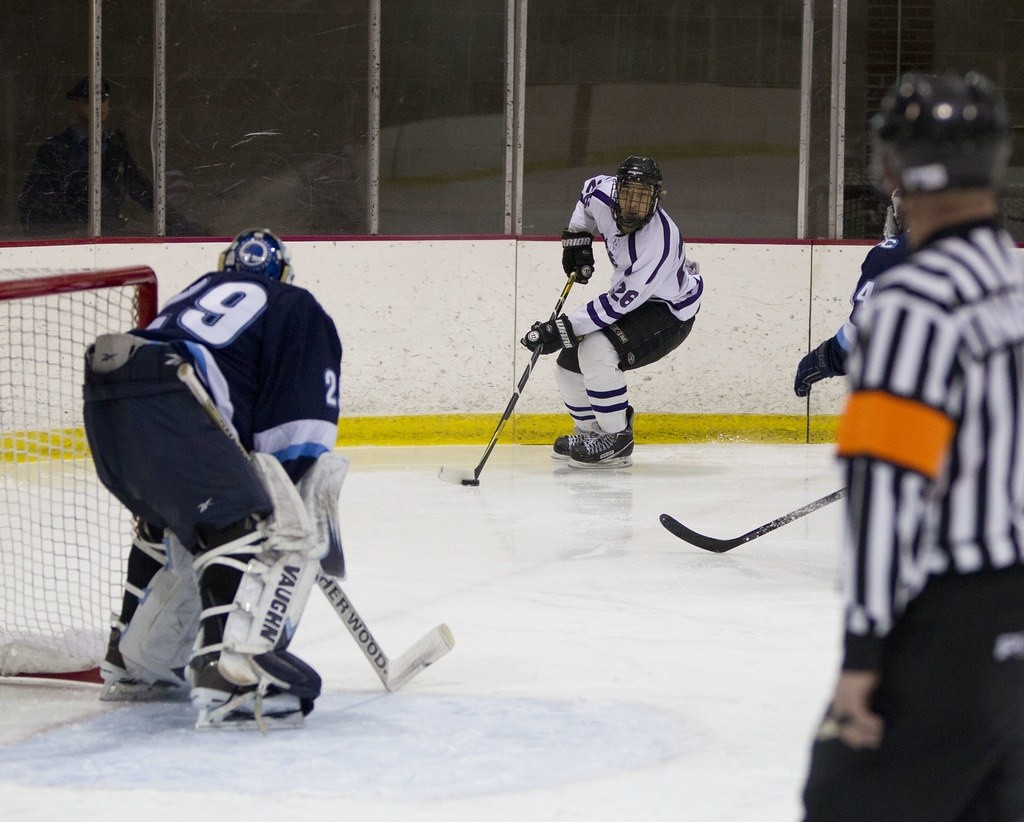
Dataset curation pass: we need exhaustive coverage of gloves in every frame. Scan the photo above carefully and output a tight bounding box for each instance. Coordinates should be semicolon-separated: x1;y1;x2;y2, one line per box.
793;335;846;398
561;227;595;285
520;312;579;355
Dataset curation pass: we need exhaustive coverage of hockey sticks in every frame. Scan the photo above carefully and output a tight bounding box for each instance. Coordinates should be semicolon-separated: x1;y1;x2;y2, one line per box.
438;264;578;484
659;486;848;554
178;361;456;693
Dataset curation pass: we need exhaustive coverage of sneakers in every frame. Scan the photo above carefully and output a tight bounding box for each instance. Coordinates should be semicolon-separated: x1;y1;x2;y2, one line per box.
567;406;634;470
98;638;190;701
551;424;599;460
190;659;301;731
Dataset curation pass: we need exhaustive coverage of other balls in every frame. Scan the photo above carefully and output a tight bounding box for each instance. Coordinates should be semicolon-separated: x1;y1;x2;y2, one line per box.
462;479;480;486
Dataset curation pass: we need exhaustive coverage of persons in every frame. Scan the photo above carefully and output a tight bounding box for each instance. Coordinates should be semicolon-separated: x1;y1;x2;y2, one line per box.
521;155;703;468
794;67;1024;822
20;79;211;235
83;228;341;722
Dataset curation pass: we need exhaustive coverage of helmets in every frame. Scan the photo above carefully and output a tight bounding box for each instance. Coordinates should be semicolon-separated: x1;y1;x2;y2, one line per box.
610;155;663;229
869;66;1010;196
217;228;294;286
